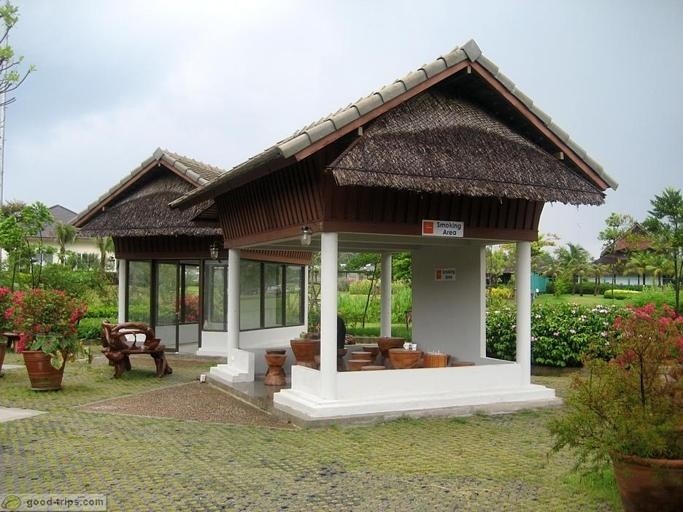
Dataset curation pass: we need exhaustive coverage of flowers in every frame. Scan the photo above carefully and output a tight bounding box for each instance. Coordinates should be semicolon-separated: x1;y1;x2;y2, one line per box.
0;287;15;344
7;286;91;374
548;298;683;482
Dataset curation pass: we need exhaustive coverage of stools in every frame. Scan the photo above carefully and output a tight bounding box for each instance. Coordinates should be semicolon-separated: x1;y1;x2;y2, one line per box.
262;333;475;394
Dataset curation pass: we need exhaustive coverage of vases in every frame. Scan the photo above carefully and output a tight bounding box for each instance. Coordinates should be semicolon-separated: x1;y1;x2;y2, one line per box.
19;348;65;388
608;454;683;511
0;343;8;377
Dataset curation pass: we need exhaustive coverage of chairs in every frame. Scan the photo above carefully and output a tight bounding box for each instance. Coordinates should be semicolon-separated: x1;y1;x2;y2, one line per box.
100;318;173;379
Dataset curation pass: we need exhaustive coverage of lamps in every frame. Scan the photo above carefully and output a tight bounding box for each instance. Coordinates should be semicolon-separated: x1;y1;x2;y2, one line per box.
209;235;222;264
300;216;317;246
